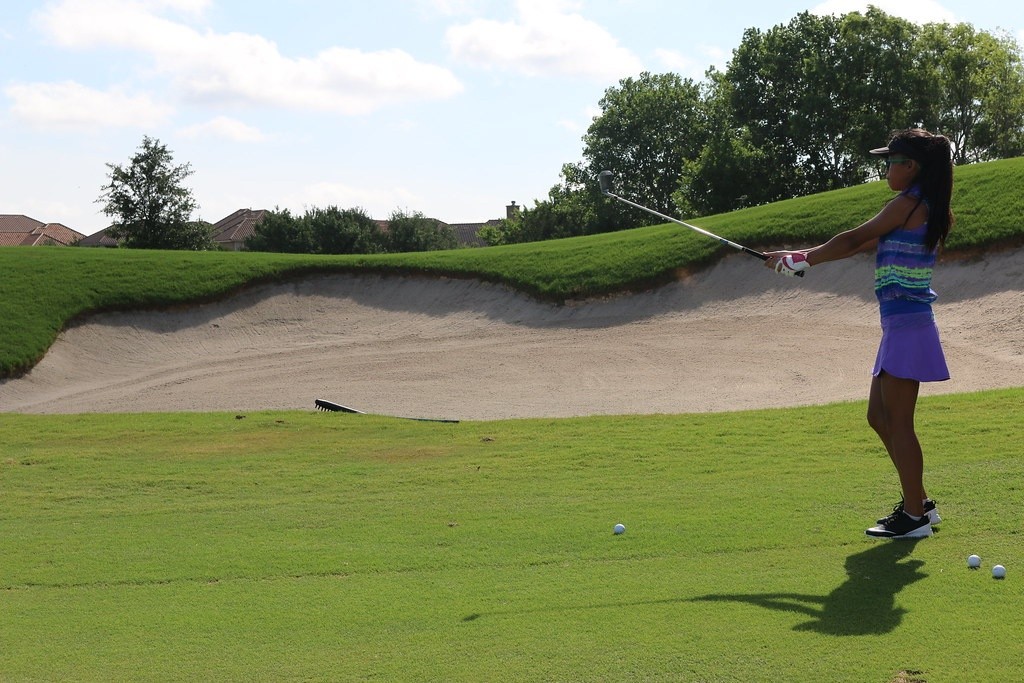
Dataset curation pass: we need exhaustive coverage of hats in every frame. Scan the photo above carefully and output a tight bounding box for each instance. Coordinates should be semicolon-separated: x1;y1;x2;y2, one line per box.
869;136;931;155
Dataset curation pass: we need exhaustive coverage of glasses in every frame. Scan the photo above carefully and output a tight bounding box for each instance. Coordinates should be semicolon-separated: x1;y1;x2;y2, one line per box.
884;158;910;165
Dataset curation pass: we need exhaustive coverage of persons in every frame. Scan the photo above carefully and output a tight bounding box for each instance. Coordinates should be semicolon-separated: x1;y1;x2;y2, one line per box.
763;128;953;538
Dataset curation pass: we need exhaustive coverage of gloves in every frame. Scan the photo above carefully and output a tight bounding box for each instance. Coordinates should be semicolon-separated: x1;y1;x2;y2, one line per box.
775;252;813;277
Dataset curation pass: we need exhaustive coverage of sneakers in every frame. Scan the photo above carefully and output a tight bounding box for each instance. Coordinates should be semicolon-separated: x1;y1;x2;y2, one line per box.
865;510;932;538
876;499;943;526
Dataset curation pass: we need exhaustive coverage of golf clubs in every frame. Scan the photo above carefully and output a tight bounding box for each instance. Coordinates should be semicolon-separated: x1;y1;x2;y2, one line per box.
599;170;805;277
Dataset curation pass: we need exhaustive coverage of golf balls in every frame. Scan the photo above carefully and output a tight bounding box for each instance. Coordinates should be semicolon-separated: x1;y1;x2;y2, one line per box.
992;565;1006;579
613;524;625;534
968;554;981;567
